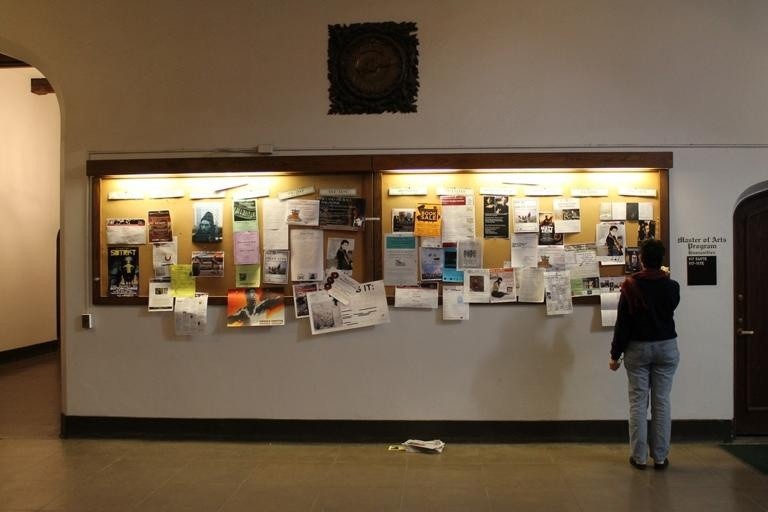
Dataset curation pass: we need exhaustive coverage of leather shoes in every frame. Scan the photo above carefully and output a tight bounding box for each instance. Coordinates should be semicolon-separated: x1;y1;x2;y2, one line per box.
629;457;668;470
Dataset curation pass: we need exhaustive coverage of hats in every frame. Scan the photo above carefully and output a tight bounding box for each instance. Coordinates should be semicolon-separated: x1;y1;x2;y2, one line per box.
201;212;213;225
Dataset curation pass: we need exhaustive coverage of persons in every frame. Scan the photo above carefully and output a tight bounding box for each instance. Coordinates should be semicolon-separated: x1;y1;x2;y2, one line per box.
496;197;508;213
336;240;352;276
192;212;222;241
606;225;623;256
484;197;494;213
491;277;502;296
609;238;681;471
